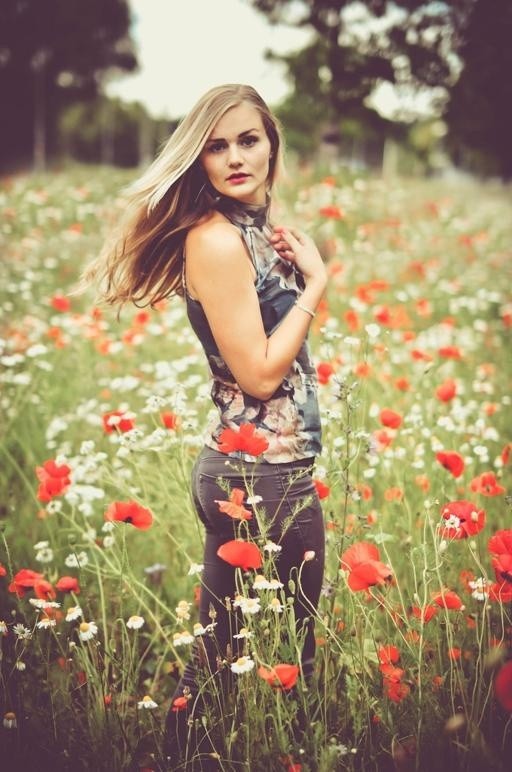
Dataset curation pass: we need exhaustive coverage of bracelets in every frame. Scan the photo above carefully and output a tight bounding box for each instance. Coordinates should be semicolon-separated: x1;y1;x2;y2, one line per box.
292;298;317;319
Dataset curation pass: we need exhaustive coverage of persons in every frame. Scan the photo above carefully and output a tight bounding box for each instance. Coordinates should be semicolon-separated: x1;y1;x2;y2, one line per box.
64;82;330;761
